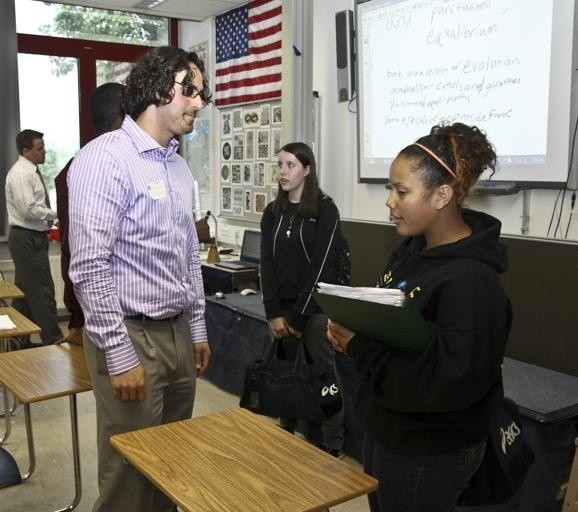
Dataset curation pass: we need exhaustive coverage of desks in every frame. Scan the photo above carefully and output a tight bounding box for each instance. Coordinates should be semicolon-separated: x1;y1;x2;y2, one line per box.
195;249;260;294
111;409;381;510
0;283;25;304
1;342;94;511
1;307;41;416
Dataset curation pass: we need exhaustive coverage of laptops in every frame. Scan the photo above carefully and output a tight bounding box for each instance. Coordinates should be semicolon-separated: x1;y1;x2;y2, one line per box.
215;229;262;271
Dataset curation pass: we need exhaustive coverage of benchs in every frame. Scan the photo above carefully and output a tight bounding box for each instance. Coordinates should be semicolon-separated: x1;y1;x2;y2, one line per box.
195;219;577;511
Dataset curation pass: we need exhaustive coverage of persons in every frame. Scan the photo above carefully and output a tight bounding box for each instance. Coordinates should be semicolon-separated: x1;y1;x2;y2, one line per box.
4;129;64;352
326;122;512;512
259;142;346;459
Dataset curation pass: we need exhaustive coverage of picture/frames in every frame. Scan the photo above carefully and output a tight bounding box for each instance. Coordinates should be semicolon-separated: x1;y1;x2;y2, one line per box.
218;104;282;217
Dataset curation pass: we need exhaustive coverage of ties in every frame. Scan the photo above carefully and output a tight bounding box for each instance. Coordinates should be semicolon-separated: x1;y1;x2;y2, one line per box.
36;165;53;227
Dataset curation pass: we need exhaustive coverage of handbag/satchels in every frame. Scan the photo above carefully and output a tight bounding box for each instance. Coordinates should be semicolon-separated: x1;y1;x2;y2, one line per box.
239;338;343;422
457;396;534;507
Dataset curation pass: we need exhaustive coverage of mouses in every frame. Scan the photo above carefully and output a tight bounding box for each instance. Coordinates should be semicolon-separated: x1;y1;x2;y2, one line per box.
241;289;257;295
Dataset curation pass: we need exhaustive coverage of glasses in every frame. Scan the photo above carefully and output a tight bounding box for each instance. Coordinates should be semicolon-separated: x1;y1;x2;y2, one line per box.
174;81;208;101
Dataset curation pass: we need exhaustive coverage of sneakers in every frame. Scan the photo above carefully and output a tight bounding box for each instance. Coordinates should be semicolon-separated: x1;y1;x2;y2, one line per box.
327;448;346;460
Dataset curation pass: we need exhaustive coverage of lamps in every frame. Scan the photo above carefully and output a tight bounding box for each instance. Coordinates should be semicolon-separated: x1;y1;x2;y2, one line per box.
195;209;224;263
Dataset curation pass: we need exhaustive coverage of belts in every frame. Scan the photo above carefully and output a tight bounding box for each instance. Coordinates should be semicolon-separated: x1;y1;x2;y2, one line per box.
124;309;183;321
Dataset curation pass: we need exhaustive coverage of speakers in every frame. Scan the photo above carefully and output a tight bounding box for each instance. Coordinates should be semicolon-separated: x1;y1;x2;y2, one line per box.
333;8;355;104
